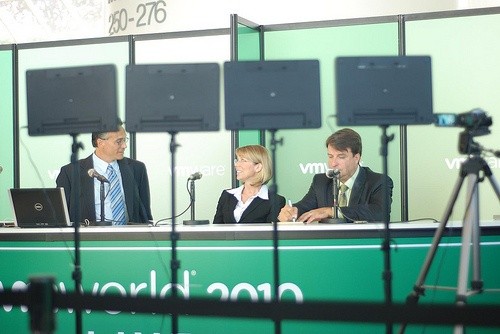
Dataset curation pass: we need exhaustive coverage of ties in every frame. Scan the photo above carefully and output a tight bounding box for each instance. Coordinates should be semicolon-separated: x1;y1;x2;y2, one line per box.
337;184;348;219
107;163;126;225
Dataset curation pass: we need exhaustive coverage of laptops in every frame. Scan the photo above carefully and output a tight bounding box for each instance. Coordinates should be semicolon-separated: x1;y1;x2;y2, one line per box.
9;187;85;228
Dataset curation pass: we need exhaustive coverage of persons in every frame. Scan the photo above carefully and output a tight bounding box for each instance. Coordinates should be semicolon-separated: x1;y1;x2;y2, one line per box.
277;128;394;224
212;145;286;223
56;118;154;228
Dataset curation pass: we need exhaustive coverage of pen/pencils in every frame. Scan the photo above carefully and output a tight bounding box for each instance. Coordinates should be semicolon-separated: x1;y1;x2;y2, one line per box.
288;200;296;222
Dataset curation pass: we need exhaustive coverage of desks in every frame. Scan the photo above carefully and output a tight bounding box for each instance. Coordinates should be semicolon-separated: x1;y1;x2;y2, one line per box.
0;222;500;334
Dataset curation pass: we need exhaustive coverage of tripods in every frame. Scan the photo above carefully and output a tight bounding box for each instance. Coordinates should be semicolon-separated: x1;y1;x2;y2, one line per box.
396;155;500;334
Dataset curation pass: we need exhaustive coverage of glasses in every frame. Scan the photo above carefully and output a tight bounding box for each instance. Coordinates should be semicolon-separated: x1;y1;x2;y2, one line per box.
98;137;128;146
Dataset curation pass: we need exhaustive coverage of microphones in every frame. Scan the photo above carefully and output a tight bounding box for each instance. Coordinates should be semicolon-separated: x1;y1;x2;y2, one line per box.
326;168;340;179
87;169;109;183
189;171;202;180
